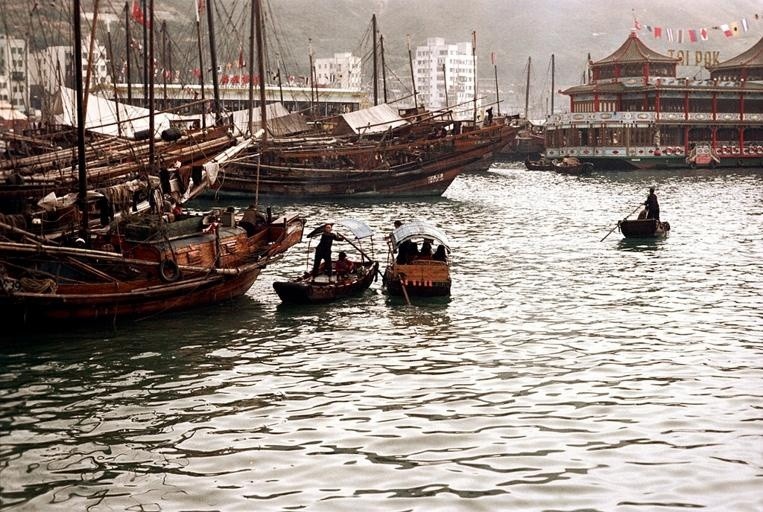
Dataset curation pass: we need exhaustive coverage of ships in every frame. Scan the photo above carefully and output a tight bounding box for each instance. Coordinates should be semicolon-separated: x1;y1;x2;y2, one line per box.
542;31;763;170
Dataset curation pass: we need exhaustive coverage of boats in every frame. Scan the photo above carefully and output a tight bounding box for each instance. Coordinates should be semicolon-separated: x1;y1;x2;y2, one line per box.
525;156;552;171
551;158;594;174
617;219;671;238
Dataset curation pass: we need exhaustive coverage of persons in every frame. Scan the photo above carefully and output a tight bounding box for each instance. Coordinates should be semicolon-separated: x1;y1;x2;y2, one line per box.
395;240;414;264
334;250;352;282
383;219;419;256
225;205;237;228
638;187;659;220
420;242;431;256
310;222;344;284
431;244;447;261
238;204;267;238
201;213;217;238
172;203;191;221
636;205;649;219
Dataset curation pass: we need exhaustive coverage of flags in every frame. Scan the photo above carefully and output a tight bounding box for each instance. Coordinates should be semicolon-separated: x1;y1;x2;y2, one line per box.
128;37;259;89
195;0;206;25
131;1;150;29
633;9;762;46
